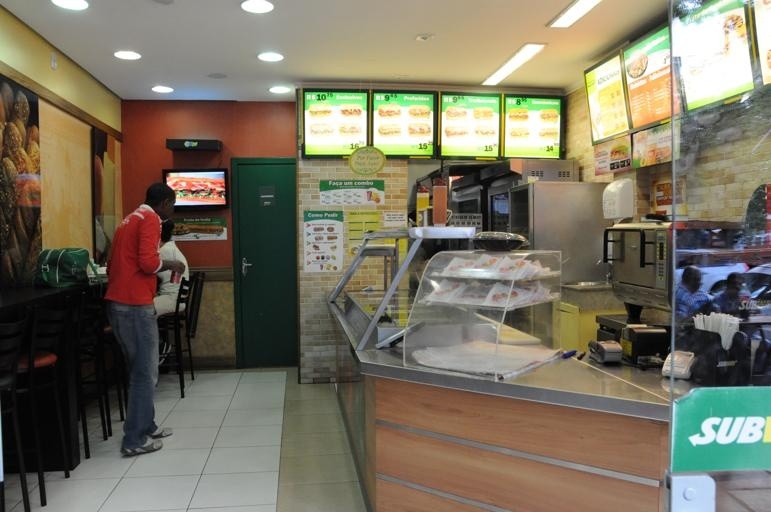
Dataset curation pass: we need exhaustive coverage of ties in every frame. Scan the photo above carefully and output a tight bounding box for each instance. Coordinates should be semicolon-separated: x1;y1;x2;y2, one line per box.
163;168;230;210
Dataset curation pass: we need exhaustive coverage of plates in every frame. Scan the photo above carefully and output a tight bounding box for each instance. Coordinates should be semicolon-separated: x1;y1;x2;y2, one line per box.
328;226;560;381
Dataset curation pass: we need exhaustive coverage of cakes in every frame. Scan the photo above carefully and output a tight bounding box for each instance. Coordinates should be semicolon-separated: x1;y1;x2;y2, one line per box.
169;270;182;284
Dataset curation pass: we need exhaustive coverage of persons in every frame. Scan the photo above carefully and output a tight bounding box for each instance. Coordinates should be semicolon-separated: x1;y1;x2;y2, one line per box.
714;270;770;386
151;219;190;388
674;265;721;386
101;183;185;457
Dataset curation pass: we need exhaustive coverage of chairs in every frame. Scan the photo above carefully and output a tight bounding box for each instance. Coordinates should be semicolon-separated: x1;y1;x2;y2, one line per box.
120;436;162;457
144;425;175;440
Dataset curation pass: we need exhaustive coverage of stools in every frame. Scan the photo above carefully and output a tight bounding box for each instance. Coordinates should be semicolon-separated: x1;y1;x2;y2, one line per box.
0;263;166;459
158;270;205;398
9;303;70;506
2;314;31;512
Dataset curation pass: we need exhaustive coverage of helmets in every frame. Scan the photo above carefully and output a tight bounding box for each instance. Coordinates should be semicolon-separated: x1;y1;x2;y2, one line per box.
627;55;648;79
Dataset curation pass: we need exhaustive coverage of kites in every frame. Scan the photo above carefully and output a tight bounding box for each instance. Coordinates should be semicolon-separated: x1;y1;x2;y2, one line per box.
563;350;577;358
577;351;586;359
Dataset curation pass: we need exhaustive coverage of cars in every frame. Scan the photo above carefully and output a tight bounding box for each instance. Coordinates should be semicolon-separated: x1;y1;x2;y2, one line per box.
646;144;657;166
415;184;448;227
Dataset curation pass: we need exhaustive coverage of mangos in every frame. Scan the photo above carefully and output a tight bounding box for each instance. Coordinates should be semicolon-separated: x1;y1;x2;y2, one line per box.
166;177;226;204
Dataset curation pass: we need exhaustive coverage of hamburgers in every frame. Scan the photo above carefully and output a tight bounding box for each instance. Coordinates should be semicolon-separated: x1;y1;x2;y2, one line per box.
308;102;559;138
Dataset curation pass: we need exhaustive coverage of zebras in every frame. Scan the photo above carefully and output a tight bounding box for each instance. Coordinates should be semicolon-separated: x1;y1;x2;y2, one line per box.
602;220;743;312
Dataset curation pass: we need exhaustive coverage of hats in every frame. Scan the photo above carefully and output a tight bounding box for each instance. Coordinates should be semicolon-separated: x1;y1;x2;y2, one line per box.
674;248;770;353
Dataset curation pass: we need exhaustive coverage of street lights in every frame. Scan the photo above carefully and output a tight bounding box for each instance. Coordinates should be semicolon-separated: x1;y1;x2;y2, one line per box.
35;245;102;291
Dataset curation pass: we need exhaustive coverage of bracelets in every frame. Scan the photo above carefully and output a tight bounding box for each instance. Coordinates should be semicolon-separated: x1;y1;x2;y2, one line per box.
472;238;524;252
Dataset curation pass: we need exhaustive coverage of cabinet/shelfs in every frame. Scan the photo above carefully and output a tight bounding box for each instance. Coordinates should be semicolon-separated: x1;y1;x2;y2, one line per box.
328;226;560;381
